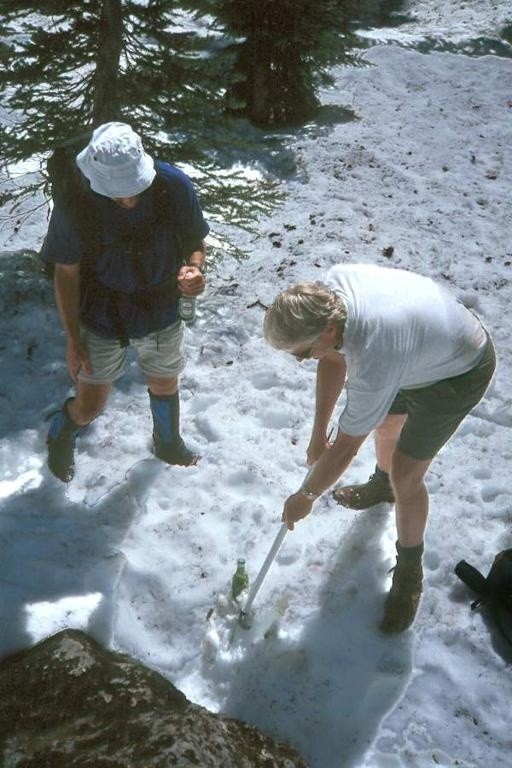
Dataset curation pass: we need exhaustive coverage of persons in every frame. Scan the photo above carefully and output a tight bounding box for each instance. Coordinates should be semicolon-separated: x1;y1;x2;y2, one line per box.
36;118;211;482
266;264;495;635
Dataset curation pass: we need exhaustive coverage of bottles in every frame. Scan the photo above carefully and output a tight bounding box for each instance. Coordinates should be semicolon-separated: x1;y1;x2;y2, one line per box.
230;559;251;602
176;273;197;325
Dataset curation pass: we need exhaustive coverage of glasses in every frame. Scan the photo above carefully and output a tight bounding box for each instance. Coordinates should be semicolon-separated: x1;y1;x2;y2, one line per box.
292;342;315;360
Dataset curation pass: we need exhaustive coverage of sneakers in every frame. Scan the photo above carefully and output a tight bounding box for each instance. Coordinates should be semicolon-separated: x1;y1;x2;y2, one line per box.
334;482;397;510
46;433;76;483
380;568;425;637
152;438;201;466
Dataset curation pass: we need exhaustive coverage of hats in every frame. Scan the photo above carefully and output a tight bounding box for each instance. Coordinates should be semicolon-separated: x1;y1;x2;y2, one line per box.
75;118;157;197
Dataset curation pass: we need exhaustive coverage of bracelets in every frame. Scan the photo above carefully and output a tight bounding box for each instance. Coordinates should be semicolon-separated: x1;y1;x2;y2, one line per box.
300;483;318;501
189;261;206;274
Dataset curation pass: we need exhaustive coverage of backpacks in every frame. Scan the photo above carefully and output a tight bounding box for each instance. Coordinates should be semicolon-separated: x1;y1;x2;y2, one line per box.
455;546;511;637
